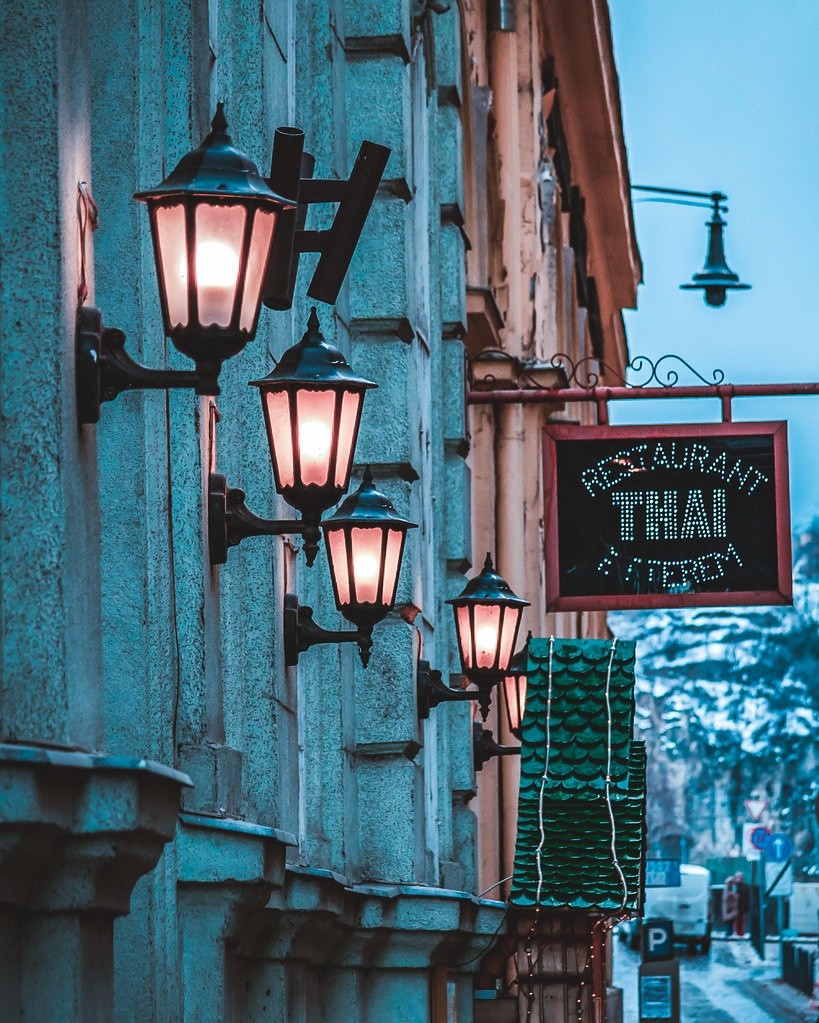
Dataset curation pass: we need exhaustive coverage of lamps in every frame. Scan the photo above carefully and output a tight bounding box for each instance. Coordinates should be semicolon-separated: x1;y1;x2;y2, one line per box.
417;551;529;720
72;99;305;427
629;183;751;308
472;630;529;772
285;462;420;673
206;305;375;573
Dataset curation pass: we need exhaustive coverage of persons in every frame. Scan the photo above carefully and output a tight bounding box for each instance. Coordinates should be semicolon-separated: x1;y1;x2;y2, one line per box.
722;871;750;936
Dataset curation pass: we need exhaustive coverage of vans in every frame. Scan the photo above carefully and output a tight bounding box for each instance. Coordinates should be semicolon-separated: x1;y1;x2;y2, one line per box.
616;863;713;957
